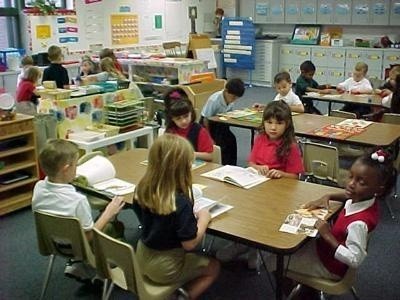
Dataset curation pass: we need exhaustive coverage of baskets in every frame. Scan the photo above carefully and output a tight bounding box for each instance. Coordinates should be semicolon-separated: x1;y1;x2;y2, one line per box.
89;80;130;92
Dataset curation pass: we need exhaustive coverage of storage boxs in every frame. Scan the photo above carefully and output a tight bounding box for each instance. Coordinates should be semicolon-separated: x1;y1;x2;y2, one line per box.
0;47;27;70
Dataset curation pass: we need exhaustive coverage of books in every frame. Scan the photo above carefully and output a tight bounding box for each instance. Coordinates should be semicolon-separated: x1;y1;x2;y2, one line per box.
72;155;137;196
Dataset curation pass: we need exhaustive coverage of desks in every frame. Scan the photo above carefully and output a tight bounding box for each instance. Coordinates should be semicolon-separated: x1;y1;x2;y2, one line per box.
71;148;344;299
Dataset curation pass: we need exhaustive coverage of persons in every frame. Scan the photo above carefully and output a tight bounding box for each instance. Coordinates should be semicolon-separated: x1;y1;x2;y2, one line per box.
133;133;221;300
31;139;126;285
15;45;144;103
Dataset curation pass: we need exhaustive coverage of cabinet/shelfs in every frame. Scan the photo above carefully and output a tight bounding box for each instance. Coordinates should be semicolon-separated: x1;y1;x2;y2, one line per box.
0;72;18;94
0;111;41;215
66;66;80;82
251;1;400;28
278;43;400;89
177;76;228;124
78;57;210;96
19;7;80;52
209;39;224;77
226;37;288;87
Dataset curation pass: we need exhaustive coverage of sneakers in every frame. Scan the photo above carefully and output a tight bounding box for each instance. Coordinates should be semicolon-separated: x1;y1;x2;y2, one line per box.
248;246;261;269
64;257;91;283
215;241;249;262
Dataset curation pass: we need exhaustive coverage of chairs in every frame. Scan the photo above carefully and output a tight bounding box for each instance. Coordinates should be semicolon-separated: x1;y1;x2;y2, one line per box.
92;227;184;300
207;87;399;220
76;151;109;210
33;210;108;299
283;232;371;299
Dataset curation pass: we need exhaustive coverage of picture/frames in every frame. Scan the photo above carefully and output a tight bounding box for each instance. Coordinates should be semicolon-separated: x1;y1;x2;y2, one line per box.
291;21;321;45
